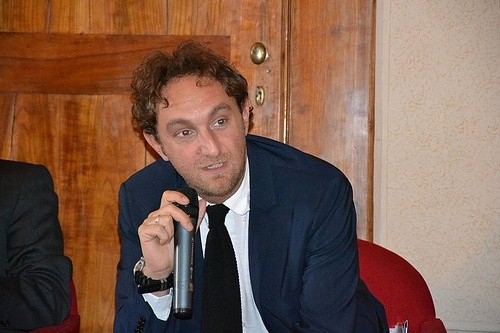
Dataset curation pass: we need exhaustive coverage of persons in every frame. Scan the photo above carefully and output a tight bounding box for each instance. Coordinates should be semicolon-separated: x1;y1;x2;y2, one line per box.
113;42;389;333
0;158;73;333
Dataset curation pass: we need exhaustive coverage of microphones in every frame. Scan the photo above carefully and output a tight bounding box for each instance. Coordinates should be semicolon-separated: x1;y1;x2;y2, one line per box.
172;188;200;321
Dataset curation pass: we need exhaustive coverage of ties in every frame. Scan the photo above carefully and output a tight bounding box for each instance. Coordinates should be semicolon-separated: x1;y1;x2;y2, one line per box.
201;204;243;333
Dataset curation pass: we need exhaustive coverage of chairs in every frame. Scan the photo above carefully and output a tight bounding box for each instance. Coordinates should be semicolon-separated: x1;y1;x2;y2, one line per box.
356;236;447;333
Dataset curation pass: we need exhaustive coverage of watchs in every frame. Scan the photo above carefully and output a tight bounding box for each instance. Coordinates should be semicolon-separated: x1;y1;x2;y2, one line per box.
133;256;175;294
